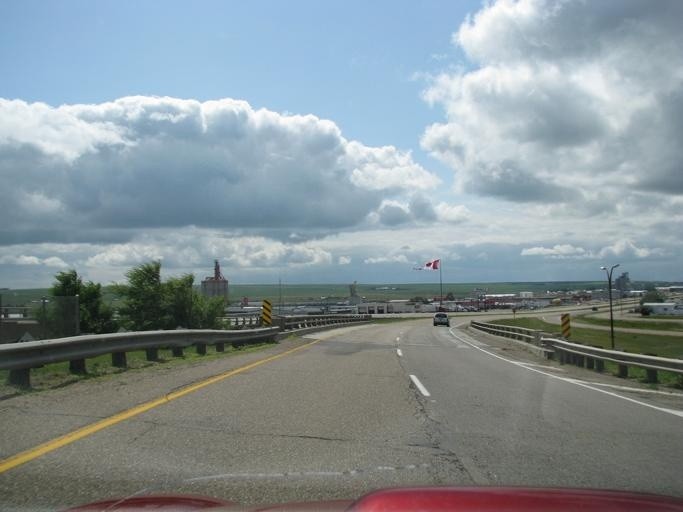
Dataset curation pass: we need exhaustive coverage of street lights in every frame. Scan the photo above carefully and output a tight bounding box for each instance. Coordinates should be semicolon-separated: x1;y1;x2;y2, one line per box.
599;264;619;348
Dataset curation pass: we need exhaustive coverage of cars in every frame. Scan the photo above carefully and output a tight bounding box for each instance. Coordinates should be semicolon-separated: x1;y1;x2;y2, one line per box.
433;312;449;326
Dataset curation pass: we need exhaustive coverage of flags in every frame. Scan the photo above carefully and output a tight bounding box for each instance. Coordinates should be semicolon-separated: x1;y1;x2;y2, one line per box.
412;258;440;271
